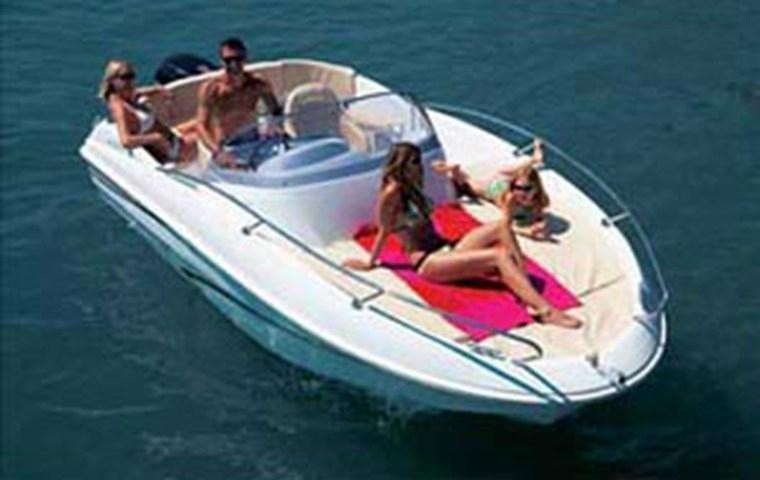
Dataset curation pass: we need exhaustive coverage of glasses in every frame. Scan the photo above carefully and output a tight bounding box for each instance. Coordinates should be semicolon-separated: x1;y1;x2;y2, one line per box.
120;74;134;80
514;185;532;191
224;56;243;63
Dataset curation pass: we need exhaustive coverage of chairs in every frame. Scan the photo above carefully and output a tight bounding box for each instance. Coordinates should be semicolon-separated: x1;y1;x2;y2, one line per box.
282;81;372;158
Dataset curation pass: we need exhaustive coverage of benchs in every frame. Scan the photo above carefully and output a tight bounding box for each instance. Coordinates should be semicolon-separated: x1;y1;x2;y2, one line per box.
136;57;357;167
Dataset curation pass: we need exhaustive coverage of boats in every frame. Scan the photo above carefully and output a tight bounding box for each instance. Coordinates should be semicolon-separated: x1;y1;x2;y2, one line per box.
78;48;669;428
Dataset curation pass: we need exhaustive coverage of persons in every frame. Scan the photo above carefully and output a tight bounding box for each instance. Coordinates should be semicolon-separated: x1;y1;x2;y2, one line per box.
98;59;201;169
196;36;290;174
431;138;552;239
344;141;584;327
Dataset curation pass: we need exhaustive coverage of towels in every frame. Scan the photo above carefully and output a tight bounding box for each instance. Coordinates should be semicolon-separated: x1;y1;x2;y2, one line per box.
350;199;583;342
